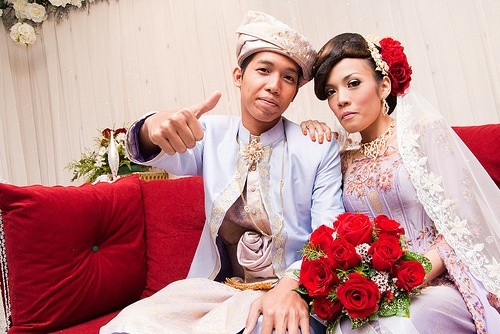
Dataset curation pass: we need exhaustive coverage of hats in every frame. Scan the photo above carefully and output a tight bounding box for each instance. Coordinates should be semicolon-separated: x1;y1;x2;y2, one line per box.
236;9;318;87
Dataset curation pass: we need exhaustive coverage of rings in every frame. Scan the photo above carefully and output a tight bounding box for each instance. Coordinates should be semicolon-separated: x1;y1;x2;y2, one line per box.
307;123;315;128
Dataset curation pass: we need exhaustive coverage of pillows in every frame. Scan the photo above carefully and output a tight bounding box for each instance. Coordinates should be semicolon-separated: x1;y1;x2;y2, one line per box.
0;174;147;334
139;176;207;300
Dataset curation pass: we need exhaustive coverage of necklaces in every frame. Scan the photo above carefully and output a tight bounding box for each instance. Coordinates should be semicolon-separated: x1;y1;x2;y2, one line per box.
237;139;288;238
359;120;395;159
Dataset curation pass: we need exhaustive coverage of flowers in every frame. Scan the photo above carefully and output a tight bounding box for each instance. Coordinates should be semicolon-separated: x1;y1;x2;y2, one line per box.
0;0;96;48
67;121;136;184
367;37;412;97
292;212;433;329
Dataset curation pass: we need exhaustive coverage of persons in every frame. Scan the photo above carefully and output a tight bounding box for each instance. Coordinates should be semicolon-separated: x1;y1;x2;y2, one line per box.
99;11;345;334
299;33;500;334
